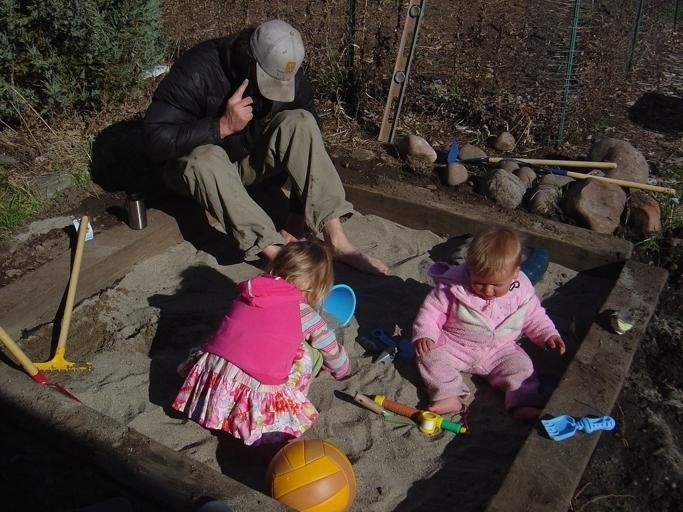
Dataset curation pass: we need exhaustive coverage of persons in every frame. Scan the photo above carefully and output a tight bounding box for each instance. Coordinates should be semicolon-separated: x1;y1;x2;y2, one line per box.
170;234;351;447
143;17;390;277
411;223;567;418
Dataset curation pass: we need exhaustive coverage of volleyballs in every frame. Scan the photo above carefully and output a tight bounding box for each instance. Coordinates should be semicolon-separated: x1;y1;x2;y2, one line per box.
263;439;356;512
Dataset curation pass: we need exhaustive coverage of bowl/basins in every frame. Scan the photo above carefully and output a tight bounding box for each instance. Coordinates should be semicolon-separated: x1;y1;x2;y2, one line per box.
322;284;355;329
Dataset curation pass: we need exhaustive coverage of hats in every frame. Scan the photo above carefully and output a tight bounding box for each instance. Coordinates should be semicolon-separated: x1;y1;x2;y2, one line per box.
247;19;307;105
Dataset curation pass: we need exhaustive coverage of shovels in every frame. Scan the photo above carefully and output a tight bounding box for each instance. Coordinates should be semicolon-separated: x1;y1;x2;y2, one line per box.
353;391;418;426
0;326;83;404
540;413;617;440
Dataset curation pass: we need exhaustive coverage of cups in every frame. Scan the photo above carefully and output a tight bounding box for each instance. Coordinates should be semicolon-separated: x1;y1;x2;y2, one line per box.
126;194;148;231
